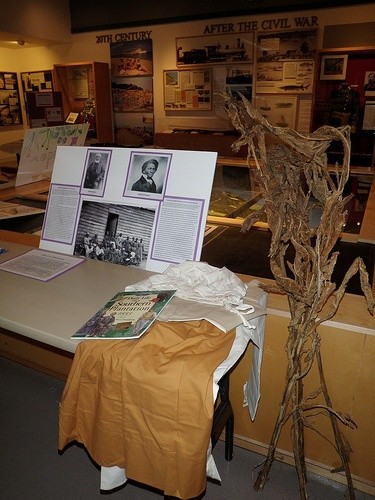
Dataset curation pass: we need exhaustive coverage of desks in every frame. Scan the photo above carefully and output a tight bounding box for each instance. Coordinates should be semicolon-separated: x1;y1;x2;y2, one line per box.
0;239;234;462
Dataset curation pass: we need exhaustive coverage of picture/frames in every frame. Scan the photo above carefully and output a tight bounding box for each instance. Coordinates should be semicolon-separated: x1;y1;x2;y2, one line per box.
254;26;319;96
320;55;347;80
175;31;253;68
162;68;212;112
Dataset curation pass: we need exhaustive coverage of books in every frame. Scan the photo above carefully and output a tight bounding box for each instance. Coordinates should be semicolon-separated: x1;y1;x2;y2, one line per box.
71;290;178;339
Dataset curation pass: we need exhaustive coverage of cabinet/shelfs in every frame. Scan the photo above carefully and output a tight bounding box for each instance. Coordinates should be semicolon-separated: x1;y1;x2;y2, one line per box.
52;61;113;144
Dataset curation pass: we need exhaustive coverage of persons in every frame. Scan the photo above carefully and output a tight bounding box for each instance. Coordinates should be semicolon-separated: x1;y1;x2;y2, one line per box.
78;153;159;266
365;73;375;91
